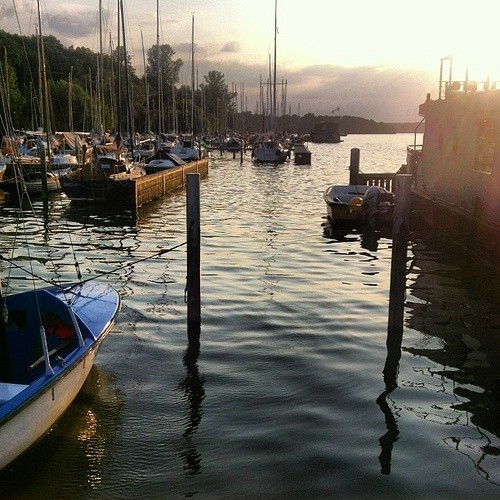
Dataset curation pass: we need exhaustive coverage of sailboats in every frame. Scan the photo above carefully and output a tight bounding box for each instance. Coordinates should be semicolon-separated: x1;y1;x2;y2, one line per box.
1;0;342;464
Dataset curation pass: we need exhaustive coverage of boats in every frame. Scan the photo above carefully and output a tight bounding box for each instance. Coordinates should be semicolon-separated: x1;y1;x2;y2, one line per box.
395;48;500;288
322;189;396;234
